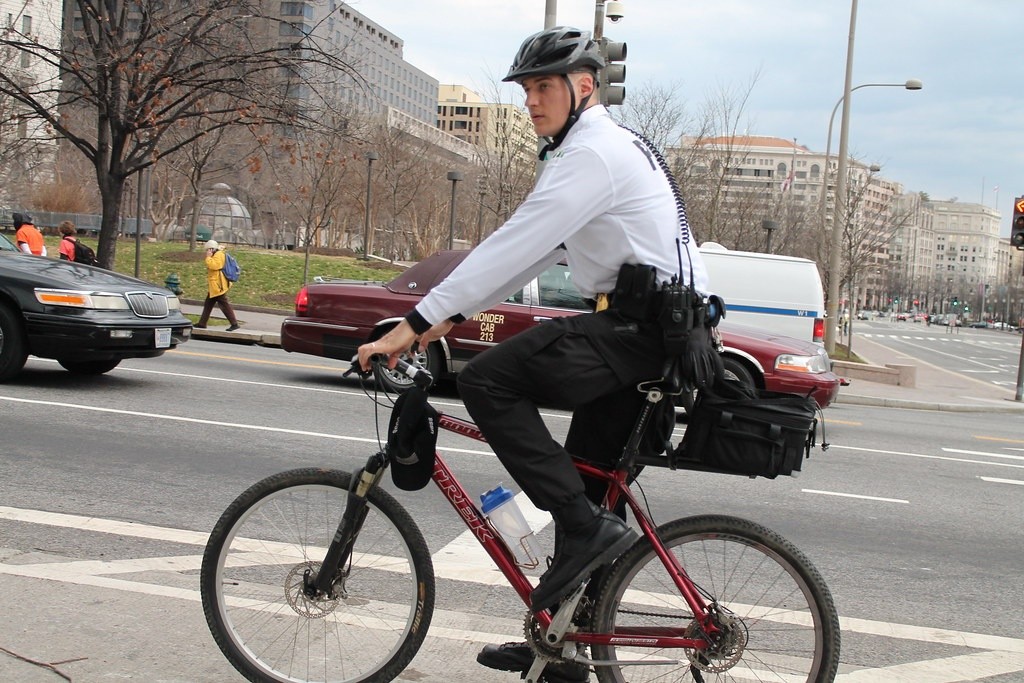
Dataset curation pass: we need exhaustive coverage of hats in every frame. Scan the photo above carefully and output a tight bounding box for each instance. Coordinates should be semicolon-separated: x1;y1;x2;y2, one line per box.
388;386;440;492
205;240;219;249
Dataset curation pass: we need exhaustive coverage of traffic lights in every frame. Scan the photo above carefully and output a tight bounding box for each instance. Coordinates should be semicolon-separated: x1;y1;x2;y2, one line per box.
1010;198;1024;247
914;299;918;305
954;297;957;305
895;297;898;304
888;296;892;302
600;37;628;107
964;305;968;312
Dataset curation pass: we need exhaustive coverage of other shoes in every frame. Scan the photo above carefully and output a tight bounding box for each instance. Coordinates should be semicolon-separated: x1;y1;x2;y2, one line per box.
226;324;242;331
193;323;207;329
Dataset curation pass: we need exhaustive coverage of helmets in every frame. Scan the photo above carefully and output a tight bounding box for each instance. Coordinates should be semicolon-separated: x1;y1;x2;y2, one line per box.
502;25;606;82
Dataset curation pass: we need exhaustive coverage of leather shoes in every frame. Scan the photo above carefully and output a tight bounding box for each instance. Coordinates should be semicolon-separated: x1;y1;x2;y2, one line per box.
529;493;640;612
477;641;590;683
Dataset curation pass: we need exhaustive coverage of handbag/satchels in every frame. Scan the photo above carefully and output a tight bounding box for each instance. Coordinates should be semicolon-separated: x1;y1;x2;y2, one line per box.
675;376;830;480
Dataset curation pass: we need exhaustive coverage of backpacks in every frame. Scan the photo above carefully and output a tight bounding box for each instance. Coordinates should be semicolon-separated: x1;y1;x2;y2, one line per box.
64;238;102;265
221;251;241;282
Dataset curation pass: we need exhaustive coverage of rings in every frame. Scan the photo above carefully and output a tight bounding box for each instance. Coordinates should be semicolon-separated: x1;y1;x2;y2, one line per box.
370;343;375;348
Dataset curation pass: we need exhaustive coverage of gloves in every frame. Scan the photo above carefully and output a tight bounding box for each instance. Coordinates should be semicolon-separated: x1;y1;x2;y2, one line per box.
662;305;725;392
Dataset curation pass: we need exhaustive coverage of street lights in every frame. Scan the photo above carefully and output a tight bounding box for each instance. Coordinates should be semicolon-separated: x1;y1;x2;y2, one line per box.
820;80;923;244
762;218;778;254
128;155;147;277
447;171;465;250
363;152;378;262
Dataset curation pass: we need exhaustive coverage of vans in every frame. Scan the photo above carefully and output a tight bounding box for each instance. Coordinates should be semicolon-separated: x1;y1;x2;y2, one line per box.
692;249;827;345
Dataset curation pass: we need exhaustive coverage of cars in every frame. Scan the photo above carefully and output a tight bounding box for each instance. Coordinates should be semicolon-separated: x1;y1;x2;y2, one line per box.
899;315;906;321
969;321;990;328
858;312;868;320
991;322;1014;329
914;317;921;322
281;251;836;412
930;315;961;326
0;233;193;382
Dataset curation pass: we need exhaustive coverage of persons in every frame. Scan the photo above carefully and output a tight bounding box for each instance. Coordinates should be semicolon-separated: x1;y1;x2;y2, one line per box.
838;317;844;336
57;220;78;262
192;239;241;332
926;314;932;327
843;309;849;336
12;212;47;257
355;25;712;683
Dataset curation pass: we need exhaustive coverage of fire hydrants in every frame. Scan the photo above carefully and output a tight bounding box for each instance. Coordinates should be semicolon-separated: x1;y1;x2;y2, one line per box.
163;272;183;298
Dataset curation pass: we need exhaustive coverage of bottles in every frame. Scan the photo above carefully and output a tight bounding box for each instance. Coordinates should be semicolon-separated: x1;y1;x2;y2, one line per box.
478;485;543;563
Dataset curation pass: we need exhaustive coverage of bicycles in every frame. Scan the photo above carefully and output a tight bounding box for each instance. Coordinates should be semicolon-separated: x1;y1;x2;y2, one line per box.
199;344;841;683
